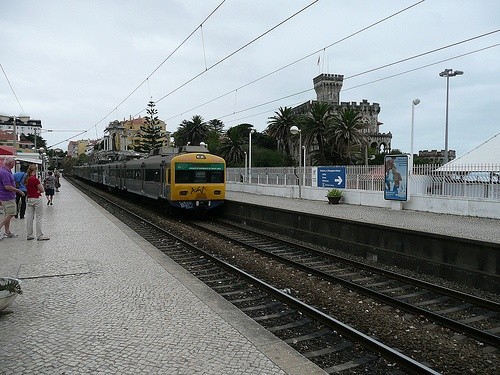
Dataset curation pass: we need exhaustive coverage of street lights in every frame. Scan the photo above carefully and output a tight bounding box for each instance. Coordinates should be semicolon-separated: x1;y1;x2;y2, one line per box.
40;147;49;182
302;146;306;186
439;69;463;163
249;130;254;183
411;99;420;177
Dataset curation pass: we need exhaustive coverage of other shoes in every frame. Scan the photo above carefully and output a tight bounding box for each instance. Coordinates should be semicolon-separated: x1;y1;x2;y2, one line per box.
4;231;18;238
26;236;35;240
0;231;3;240
37;236;50;241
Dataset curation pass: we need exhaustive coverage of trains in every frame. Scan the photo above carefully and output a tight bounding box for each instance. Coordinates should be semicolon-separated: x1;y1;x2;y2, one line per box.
72;146;226;213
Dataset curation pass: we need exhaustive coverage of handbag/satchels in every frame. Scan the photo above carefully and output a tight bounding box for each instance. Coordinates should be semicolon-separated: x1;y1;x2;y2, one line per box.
15;182;20;188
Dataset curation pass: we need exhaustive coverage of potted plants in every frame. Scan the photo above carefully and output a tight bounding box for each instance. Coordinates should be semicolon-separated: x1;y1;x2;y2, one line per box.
0;277;23;311
326;188;343;205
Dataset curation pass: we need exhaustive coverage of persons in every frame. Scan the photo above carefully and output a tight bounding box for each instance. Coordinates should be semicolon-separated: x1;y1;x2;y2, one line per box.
44;171;58;205
13;164;29;220
386;157;402;192
54;170;61;192
23;165;50;240
0;157;25;239
